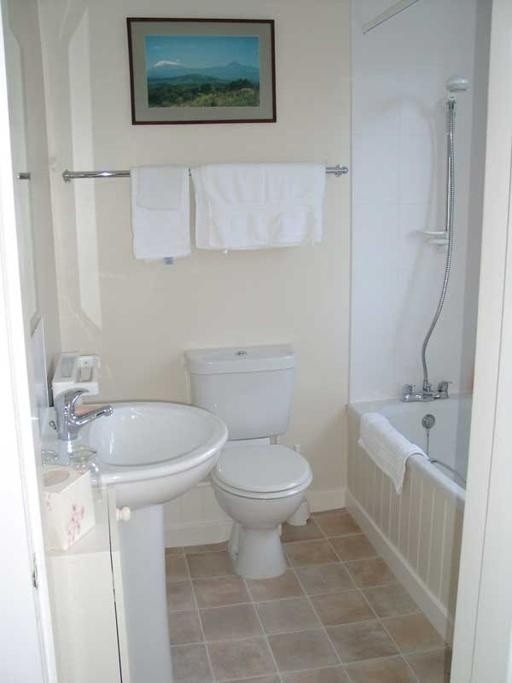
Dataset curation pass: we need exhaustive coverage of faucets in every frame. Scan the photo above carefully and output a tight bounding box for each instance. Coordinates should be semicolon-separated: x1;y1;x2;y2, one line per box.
51;386;113;439
421;381;442;403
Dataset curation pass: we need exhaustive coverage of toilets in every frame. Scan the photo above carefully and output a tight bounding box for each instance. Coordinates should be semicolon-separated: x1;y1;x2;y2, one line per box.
187;347;312;580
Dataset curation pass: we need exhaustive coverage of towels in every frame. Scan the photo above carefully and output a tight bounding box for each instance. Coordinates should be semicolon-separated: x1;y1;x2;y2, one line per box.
192;163;327;251
356;410;429;493
129;164;192;265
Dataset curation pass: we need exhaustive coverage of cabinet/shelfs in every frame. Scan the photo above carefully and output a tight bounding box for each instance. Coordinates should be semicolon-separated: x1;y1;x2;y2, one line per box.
46;486;130;683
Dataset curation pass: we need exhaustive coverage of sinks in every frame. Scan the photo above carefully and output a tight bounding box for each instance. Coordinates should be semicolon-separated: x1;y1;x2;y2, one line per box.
44;401;229;511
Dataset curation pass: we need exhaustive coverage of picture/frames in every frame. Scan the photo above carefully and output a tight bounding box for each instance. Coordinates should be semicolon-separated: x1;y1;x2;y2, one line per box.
127;17;277;124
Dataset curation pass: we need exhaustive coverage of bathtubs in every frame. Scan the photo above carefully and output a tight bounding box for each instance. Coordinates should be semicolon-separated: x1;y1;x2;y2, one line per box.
346;393;469;514
